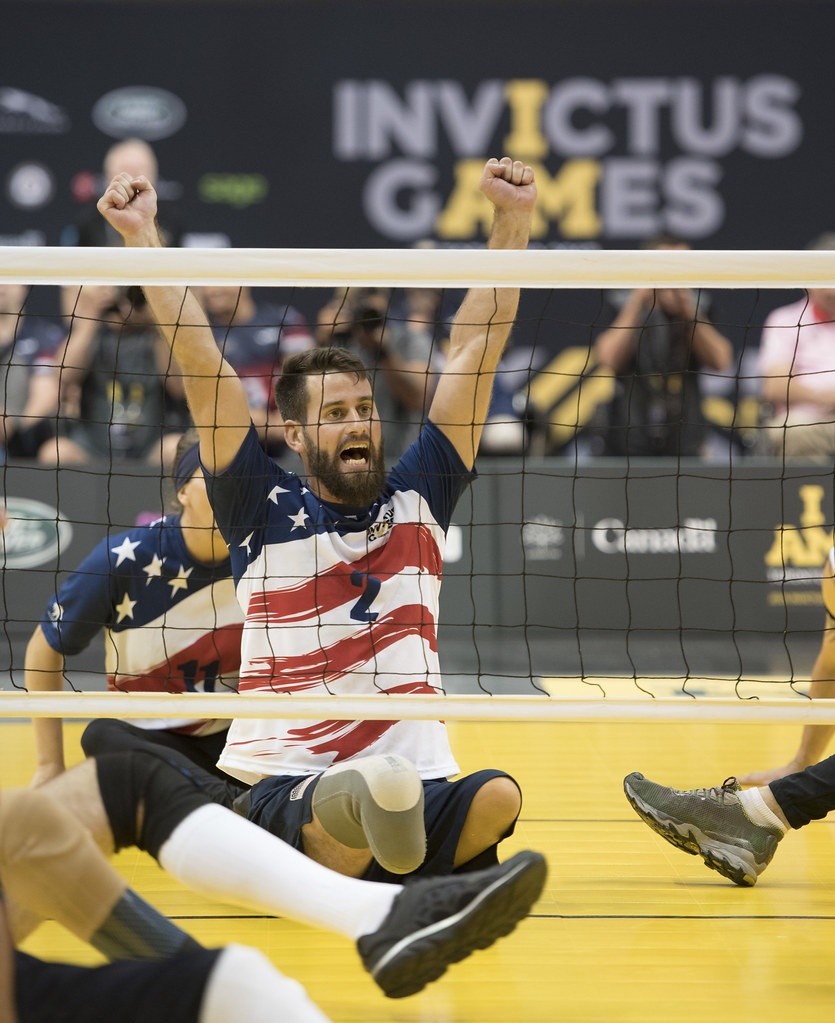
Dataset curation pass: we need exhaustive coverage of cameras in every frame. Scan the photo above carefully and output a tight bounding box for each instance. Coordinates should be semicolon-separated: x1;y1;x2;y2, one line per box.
103;285;146;315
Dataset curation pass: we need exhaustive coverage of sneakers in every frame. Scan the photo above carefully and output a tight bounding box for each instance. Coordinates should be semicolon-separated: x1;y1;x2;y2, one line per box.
625;772;791;887
354;849;548;998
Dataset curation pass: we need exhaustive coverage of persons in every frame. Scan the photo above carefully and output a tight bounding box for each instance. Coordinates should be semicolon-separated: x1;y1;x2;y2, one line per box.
96;157;536;882
1;137;529;456
737;551;834;786
0;750;547;1023
590;242;730;456
622;754;835;887
24;427;246;791
756;233;834;461
